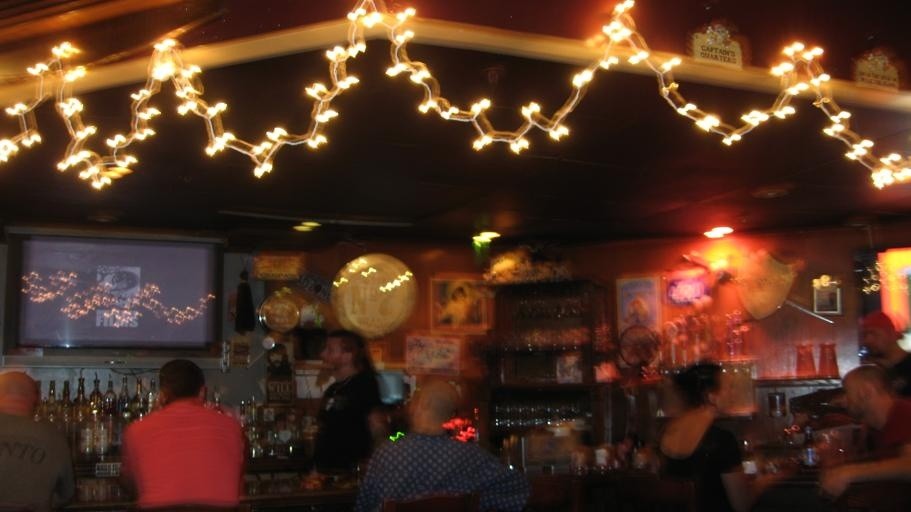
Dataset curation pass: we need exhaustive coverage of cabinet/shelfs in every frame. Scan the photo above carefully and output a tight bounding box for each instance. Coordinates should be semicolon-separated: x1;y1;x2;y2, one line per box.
484;278;608;456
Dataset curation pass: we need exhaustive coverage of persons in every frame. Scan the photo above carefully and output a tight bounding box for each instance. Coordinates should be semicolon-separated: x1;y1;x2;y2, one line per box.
1;370;81;512
816;360;910;511
123;359;251;511
298;326;387;489
351;377;530;512
656;359;751;511
856;310;910;398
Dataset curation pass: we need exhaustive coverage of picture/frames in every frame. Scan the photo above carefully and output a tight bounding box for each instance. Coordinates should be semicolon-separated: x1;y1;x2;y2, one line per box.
813;285;841;314
613;272;660;370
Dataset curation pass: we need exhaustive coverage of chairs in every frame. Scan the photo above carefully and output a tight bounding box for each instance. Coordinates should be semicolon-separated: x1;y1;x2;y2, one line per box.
518;475;572;512
631;473;697;511
379;490;474;512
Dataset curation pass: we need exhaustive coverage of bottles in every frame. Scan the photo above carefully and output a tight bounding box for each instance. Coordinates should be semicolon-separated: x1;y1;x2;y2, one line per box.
803;427;818;470
132;377;146;416
47;381;59;424
145;379;159;415
95;415;108;456
91;379;103;416
80;414;94;458
60;381;73;421
103;379;116;415
75;379;89;421
118;375;131;414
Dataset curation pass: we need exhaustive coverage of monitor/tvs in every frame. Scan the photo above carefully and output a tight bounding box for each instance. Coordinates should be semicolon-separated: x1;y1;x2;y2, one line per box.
5;227;227;371
